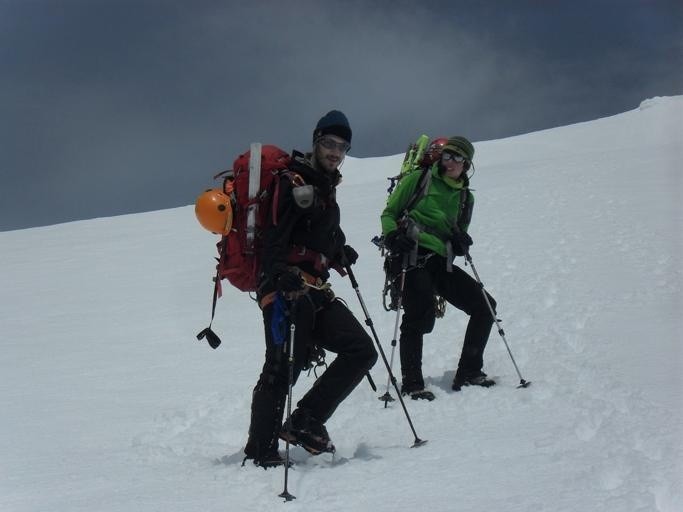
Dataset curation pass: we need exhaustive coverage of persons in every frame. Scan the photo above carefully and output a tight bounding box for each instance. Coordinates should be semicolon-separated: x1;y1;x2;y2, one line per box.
244;110;378;467
381;135;496;400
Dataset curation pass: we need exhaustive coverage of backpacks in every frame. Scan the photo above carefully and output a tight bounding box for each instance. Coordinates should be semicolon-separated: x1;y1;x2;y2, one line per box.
218;143;291;292
395;135;429;184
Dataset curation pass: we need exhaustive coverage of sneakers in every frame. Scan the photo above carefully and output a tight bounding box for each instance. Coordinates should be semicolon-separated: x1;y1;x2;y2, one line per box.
454;370;495;388
244;438;287;463
401;390;434;401
284;410;334;452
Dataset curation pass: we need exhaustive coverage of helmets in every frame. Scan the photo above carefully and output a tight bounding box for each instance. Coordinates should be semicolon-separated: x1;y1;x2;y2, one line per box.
195;189;233;237
430;139;448;149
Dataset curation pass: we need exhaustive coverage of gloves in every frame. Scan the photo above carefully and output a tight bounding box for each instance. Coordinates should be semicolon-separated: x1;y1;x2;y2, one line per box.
455;232;473;256
337;245;358;266
386;230;416;253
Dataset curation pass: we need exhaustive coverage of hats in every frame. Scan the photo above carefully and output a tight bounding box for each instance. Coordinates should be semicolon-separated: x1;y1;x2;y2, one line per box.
313;110;351;143
441;137;474;170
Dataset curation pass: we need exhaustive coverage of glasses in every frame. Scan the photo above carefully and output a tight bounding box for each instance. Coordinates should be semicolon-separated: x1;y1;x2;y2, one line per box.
442;151;466;163
321;139;349;153
